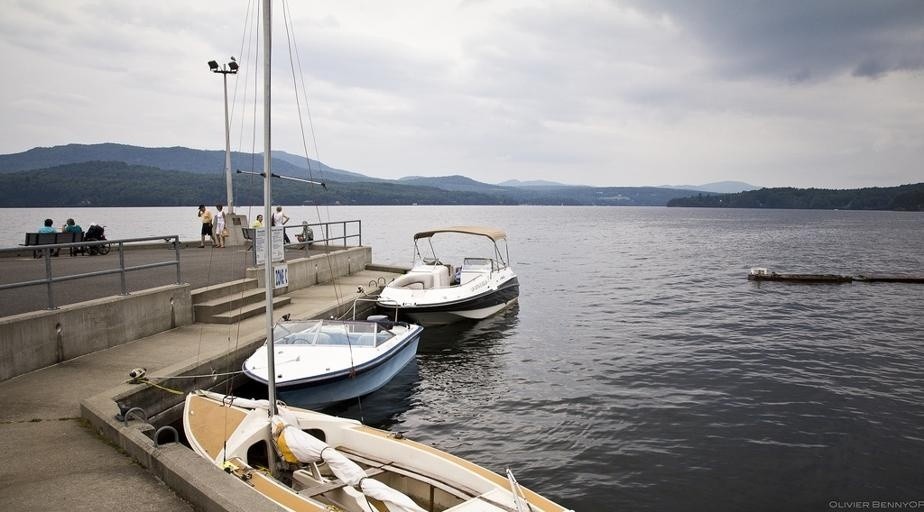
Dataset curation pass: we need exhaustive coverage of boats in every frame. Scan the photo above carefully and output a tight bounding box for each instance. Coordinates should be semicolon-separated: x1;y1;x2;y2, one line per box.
238;303;430;415
743;265;854;285
373;223;524;329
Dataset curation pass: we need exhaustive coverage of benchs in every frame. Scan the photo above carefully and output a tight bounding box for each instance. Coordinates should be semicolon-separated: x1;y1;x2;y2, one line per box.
17;231;85;258
240;227;254;250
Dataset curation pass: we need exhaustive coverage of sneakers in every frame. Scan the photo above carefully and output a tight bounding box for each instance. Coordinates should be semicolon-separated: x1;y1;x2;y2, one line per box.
211;244;225;248
197;245;204;248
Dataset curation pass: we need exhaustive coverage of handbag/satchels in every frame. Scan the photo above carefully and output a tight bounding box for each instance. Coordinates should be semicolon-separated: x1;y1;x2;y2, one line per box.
271;213;275;227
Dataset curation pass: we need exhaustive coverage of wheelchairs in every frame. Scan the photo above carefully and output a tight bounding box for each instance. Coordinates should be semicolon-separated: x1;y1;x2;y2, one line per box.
83;223;112;258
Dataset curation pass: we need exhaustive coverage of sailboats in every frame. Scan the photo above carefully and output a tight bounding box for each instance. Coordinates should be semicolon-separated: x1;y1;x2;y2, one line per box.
176;0;576;512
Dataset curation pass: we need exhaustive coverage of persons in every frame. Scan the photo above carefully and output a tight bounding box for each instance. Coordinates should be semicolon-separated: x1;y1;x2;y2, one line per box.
53;218;82;256
273;207;290;242
38;220;56;258
213;204;226;248
253;214;263;228
294;221;313;250
198;205;216;248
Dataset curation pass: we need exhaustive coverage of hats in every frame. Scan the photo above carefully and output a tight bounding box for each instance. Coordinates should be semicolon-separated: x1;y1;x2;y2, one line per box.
198;205;204;209
302;221;308;225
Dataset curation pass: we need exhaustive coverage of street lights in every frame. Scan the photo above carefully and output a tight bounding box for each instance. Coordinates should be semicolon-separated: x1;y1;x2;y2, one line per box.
206;54;243;216
